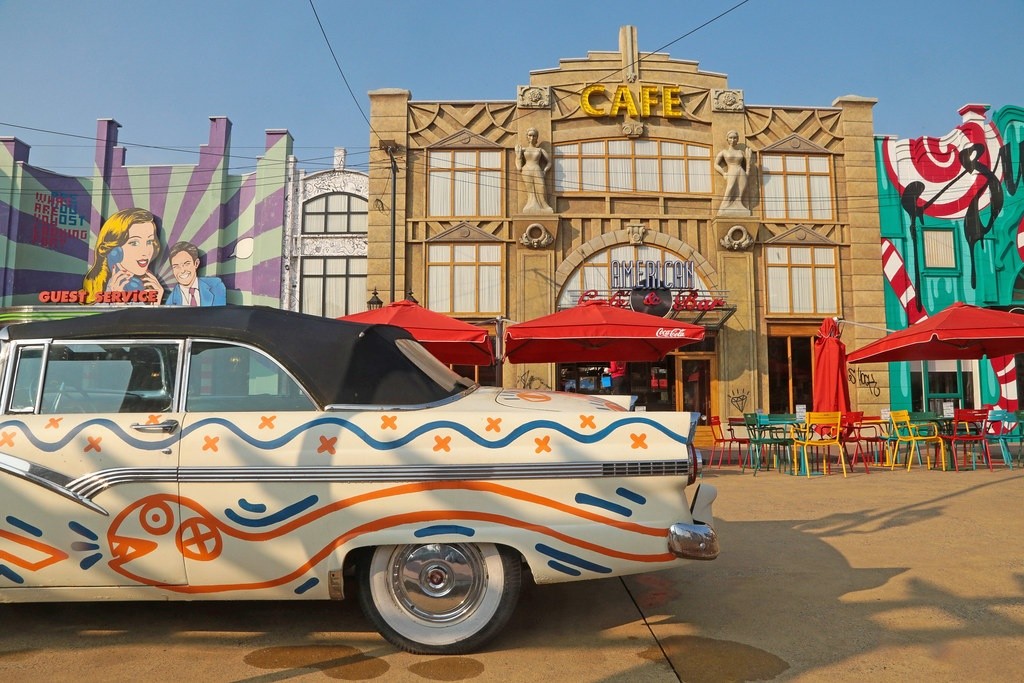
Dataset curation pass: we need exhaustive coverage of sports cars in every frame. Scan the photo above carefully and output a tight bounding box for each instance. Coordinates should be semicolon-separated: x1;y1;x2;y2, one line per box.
0;304;721;657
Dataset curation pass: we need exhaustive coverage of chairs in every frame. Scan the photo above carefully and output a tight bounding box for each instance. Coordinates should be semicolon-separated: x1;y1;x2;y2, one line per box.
702;407;1024;479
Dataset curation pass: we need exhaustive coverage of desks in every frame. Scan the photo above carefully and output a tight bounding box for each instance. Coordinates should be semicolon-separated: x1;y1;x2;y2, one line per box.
854;418;906;467
759;420;824;476
908;416;956;437
